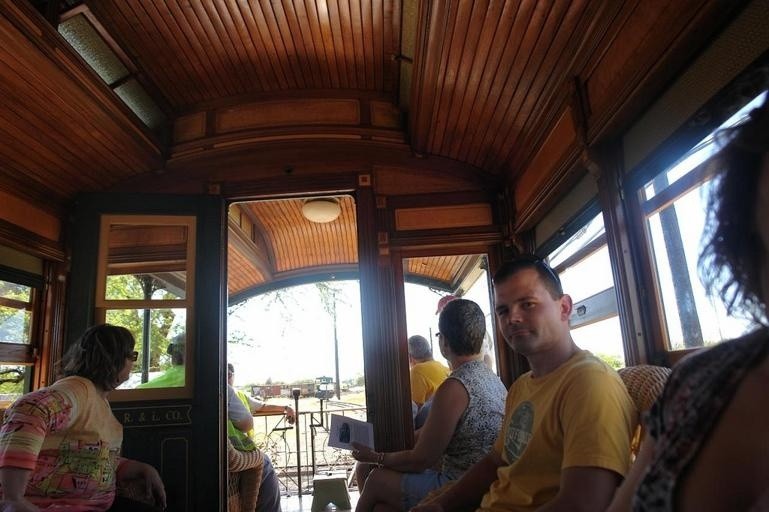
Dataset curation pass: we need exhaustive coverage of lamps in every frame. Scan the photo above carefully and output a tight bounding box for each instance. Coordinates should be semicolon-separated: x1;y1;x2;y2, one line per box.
300;195;343;227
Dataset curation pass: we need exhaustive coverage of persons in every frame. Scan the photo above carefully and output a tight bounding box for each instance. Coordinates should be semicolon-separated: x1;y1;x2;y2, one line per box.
435;296;496;375
407;335;450;427
228;363;295;446
355;297;509;511
134;332;282;511
411;256;639;511
1;322;168;510
608;90;767;510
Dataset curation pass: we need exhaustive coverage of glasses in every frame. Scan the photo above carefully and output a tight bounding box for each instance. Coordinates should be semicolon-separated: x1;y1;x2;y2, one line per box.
492;256;558;285
127;352;138;361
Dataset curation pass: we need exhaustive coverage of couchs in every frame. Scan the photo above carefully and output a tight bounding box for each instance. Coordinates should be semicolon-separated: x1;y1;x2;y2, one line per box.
227;435;266;512
615;361;676;420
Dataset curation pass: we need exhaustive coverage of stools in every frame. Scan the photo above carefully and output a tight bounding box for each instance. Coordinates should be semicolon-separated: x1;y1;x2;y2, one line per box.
310;468;354;511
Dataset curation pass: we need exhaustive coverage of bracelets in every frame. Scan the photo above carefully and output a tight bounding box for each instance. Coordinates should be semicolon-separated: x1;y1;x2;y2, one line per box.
378;452;384;468
283;405;288;415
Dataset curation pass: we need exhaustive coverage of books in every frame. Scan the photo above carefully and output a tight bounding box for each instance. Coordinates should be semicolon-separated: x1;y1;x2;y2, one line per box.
327;414;374;452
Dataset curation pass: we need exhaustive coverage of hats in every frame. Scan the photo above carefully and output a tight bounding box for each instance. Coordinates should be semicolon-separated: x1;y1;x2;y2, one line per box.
435;296;455;315
